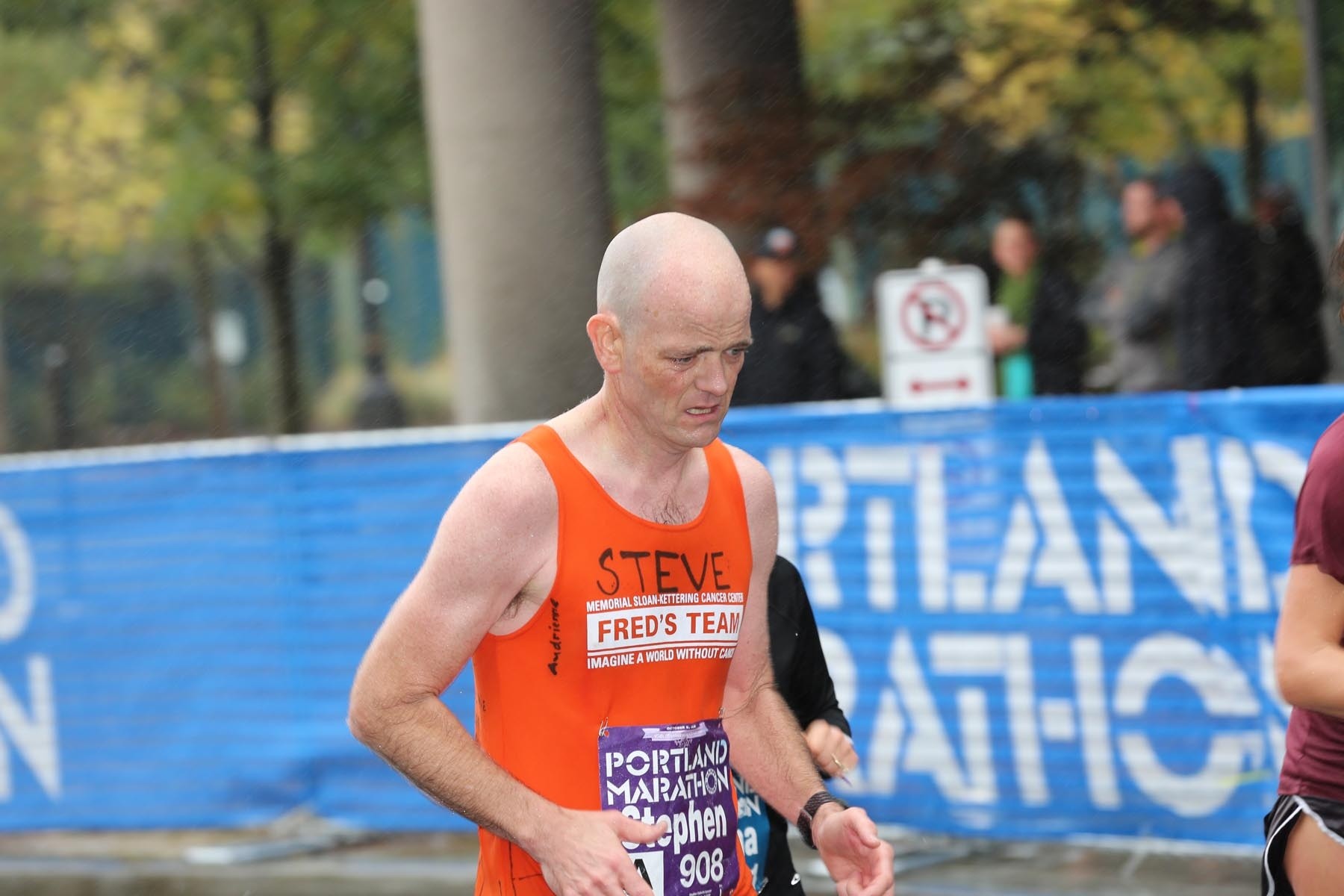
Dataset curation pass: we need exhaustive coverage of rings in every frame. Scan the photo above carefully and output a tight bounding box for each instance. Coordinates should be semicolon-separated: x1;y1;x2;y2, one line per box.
835;761;842;769
831;752;836;761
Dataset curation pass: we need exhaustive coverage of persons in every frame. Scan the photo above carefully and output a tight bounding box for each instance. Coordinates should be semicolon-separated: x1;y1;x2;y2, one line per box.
731;160;1329;407
347;213;896;895
1262;410;1344;896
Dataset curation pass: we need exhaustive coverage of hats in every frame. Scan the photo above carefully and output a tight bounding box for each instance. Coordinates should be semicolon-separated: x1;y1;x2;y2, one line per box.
757;224;803;262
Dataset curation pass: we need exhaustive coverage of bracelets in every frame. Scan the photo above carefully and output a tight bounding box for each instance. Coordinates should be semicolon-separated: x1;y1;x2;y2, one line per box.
796;790;850;849
